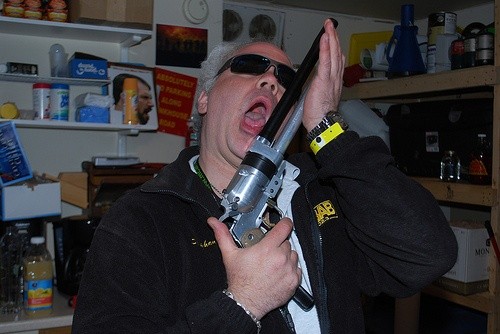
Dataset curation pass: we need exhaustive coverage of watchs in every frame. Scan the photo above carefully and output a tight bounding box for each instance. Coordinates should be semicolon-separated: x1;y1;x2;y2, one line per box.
305;111;349;145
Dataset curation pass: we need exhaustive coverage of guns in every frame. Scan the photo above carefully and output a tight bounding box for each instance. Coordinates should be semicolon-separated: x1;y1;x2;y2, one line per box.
216;17;340;313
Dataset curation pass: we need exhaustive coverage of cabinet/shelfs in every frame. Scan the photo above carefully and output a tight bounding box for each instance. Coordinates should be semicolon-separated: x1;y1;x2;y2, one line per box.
0;16;159;166
339;0;500;334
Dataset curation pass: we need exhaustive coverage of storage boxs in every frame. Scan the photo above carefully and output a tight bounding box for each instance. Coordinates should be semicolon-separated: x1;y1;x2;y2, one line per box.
435;218;489;296
0;121;62;222
5;62;39;75
68;52;108;80
75;106;111;122
65;0;155;29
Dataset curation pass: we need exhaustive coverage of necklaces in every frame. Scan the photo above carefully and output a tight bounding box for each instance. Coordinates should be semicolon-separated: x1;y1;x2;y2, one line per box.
211;185;224;197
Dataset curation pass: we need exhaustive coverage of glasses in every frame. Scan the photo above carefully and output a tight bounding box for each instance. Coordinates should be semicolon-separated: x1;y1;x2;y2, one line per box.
213;53;297;90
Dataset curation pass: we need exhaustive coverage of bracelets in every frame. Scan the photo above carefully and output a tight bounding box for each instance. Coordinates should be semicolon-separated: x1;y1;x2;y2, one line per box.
222;289;261;329
309;122;345;155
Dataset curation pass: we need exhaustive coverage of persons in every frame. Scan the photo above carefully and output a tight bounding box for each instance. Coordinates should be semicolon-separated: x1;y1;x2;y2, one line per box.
71;18;459;334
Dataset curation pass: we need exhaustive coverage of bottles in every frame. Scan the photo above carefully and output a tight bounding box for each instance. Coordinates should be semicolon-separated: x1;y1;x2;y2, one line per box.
440;150;461;184
469;134;492;185
23;236;53;319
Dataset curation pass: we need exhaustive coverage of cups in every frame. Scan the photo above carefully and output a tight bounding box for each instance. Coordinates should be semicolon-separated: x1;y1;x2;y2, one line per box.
426;11;457;74
49;83;70;122
475;32;493;66
32;84;50;121
48;43;67;77
464;34;477;68
451;39;464;70
